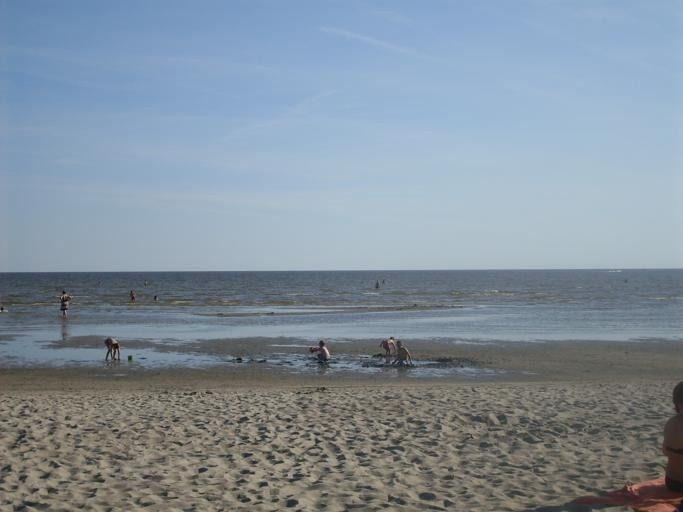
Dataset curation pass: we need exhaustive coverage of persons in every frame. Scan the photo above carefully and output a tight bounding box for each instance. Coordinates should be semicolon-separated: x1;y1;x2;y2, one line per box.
130;288;136;303
392;341;412;366
144;280;147;288
60;289;72;319
310;340;330;361
0;307;8;313
664;383;683;490
375;279;381;289
104;337;120;361
379;337;395;356
